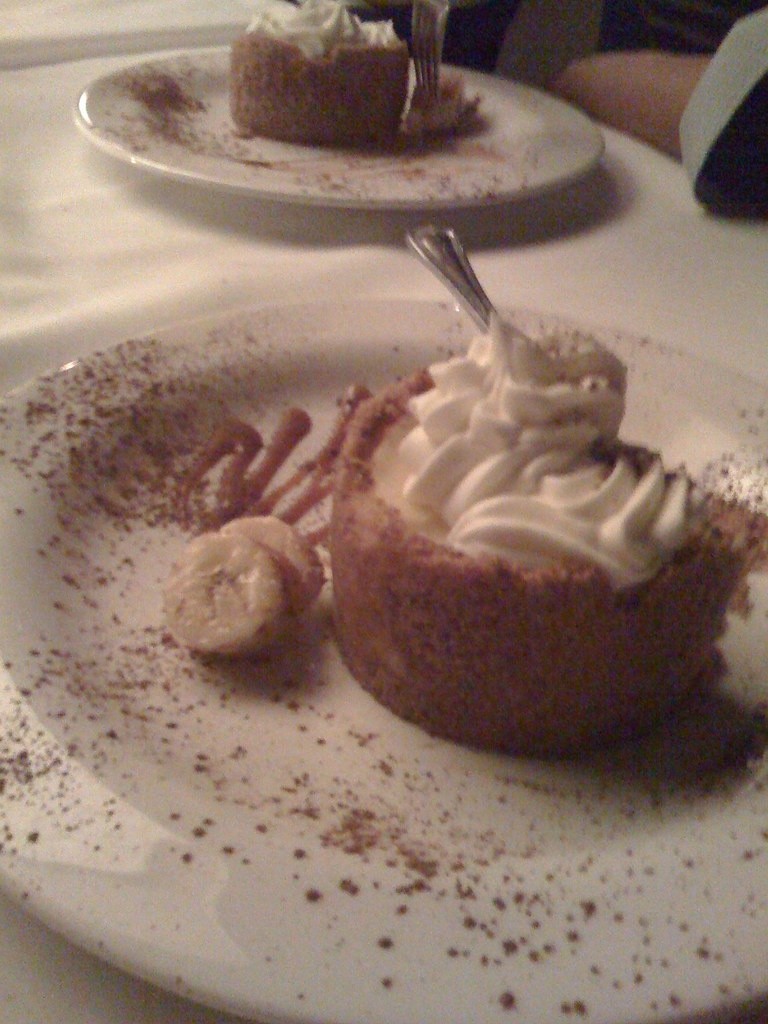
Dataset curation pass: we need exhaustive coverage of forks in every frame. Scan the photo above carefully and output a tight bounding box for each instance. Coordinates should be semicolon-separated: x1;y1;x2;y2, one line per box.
409;0;450;101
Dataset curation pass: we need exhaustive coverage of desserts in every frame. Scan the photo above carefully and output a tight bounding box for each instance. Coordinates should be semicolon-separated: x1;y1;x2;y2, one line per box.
328;316;735;757
227;0;410;143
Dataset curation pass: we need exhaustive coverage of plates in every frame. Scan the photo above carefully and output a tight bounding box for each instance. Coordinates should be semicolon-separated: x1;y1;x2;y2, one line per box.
0;300;768;1024
77;51;605;209
